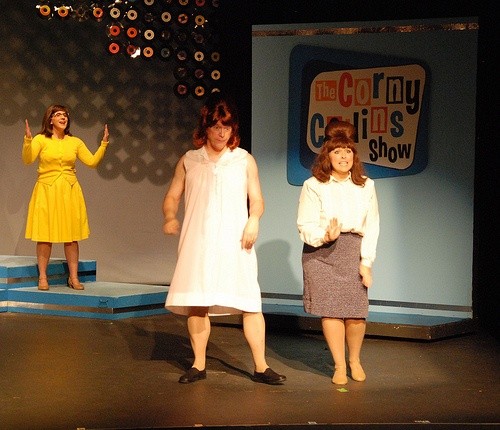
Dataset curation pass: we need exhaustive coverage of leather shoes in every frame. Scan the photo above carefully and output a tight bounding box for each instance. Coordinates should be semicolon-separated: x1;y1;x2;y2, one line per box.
179;368;206;383
253;368;287;384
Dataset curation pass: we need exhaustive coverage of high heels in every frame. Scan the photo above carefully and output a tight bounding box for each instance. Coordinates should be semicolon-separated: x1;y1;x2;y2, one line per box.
38;280;49;290
348;361;366;382
331;365;348;385
68;278;84;290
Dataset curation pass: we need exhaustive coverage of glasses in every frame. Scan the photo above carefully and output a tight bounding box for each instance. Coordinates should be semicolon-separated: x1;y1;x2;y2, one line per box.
51;113;68;117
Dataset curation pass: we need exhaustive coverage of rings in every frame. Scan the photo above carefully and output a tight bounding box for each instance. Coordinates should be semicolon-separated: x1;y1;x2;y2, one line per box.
108;133;109;135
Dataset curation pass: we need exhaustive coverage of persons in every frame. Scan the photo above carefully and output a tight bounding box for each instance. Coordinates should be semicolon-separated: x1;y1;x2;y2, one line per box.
297;121;380;385
163;99;286;385
22;104;109;291
